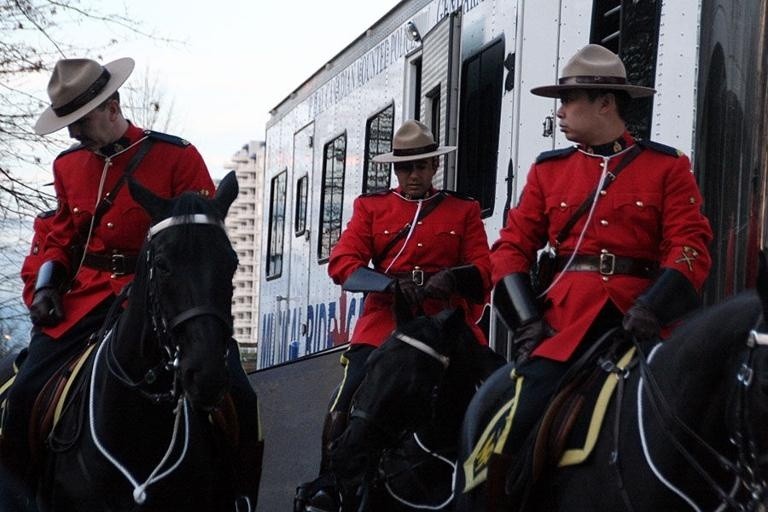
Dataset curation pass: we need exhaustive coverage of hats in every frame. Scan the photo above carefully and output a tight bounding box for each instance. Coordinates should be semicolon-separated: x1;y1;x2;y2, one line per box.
373;119;458;164
531;44;658;98
34;57;135;136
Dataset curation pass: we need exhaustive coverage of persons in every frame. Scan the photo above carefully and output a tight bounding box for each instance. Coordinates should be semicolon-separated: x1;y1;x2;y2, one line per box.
455;43;713;512
0;55;218;511
308;119;490;507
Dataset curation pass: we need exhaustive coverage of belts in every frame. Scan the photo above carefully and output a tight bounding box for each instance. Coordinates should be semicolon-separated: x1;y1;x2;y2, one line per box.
556;252;661;279
425;270;453;300
392;270;438;289
82;253;138;277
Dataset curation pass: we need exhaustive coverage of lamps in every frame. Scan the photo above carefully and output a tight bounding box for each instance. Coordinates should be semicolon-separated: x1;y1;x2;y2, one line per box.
542;115;554;138
405;20;423;47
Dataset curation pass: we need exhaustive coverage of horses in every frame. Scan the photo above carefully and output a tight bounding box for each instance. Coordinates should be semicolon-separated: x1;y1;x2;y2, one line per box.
290;277;768;512
0;165;266;512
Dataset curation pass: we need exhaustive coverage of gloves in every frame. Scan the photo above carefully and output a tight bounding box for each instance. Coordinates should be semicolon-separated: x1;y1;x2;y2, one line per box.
30;289;67;330
513;320;556;370
622;306;654;336
398;279;426;307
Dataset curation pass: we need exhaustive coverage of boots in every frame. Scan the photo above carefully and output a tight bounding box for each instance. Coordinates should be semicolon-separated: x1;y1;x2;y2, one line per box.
301;410;352;510
